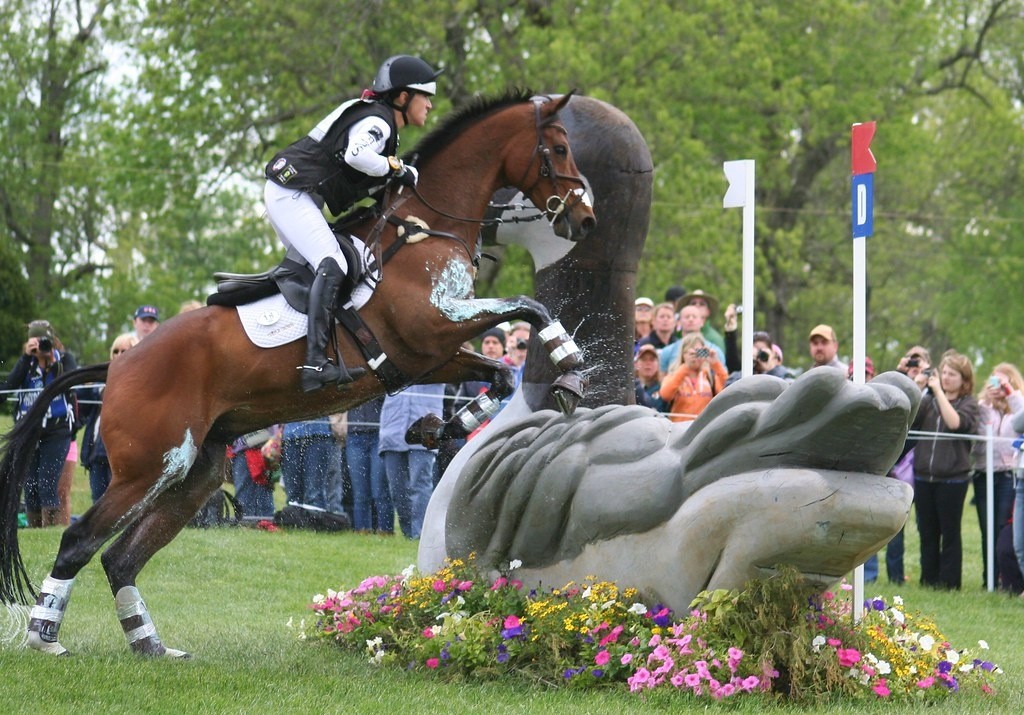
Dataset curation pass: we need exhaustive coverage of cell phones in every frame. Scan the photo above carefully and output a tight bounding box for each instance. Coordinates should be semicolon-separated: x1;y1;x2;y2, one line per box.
695;349;709;357
990;376;1000;388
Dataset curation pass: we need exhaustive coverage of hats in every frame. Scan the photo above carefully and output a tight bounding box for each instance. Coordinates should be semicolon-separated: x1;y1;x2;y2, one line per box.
508;321;532;333
849;356;874;374
665;285;685;302
906;345;931;364
808;324;837;341
637;343;659;360
480;327;505;346
28;324;54;340
496;322;511;331
676;289;719;320
772;344;783;361
133;304;159;321
635;296;654;308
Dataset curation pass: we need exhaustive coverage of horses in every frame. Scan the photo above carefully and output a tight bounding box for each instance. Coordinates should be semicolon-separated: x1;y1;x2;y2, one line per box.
0;84;597;661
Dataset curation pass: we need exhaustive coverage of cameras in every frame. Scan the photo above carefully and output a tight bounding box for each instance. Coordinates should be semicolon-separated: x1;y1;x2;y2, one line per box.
516;338;529;350
923;370;932;377
906;360;919;367
735;305;742;314
757;350;768;361
32;337;53;354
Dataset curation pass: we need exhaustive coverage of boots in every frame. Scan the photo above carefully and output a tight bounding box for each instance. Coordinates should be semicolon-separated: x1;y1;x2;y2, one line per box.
302;257;367;393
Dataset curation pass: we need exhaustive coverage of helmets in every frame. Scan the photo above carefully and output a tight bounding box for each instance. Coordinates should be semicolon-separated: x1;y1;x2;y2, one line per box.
372;54;445;96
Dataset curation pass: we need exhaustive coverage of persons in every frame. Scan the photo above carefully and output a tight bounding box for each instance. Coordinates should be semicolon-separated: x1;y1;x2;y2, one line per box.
7;302;531;539
265;54;447;391
634;288;1024;598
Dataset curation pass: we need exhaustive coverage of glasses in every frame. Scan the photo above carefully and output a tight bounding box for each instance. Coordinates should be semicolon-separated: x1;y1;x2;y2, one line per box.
29;319;49;329
637;306;651;312
112;349;126;354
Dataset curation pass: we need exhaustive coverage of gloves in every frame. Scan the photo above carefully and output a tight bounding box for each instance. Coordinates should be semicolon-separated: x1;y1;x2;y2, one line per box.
383;157;419;187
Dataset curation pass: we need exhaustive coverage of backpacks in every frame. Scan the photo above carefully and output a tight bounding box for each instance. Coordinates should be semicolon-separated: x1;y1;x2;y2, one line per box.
272;499;352;533
